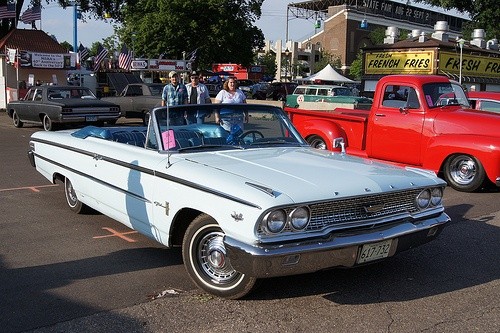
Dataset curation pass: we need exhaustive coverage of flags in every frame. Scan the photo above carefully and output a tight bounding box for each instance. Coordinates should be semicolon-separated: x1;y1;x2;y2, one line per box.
21;5;40;24
119;43;132;70
94;44;109;71
0;4;15;18
79;44;90;61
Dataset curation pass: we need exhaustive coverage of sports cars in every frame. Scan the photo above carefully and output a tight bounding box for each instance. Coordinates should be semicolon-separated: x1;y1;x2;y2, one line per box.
28;104;451;302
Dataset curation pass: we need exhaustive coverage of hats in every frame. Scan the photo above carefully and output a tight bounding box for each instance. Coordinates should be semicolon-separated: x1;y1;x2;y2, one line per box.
168;70;177;78
190;71;198;76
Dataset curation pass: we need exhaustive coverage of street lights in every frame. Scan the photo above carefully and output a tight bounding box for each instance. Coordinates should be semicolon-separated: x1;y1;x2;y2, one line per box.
456;38;465;86
285;47;290;82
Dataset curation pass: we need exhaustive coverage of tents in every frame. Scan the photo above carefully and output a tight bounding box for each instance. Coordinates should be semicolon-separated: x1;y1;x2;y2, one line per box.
293;63;361;86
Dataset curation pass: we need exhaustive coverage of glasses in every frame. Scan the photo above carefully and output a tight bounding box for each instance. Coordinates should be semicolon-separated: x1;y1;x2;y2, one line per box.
190;77;198;79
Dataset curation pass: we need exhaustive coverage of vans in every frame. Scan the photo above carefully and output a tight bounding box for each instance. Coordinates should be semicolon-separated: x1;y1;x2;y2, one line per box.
292;85;354;97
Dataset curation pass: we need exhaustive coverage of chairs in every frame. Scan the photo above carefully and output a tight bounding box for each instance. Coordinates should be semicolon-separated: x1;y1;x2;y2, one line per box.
188;137;226;146
155;138;191;151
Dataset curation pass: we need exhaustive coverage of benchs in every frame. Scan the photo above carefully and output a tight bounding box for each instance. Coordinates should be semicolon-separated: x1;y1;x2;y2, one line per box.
114;131;201;147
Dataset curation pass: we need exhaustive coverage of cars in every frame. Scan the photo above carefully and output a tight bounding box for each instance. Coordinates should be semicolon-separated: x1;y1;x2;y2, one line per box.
6;84;122;132
204;77;299;101
433;91;500;112
100;83;166;126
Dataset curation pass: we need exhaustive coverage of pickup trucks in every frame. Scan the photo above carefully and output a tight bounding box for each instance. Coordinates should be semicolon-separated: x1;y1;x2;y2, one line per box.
281;74;500;193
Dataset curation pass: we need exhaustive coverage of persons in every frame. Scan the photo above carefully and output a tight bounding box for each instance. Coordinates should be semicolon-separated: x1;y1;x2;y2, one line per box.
36;80;42;86
185;71;212;123
72;77;80;86
352;86;359;96
215;77;248;132
162;71;189;126
